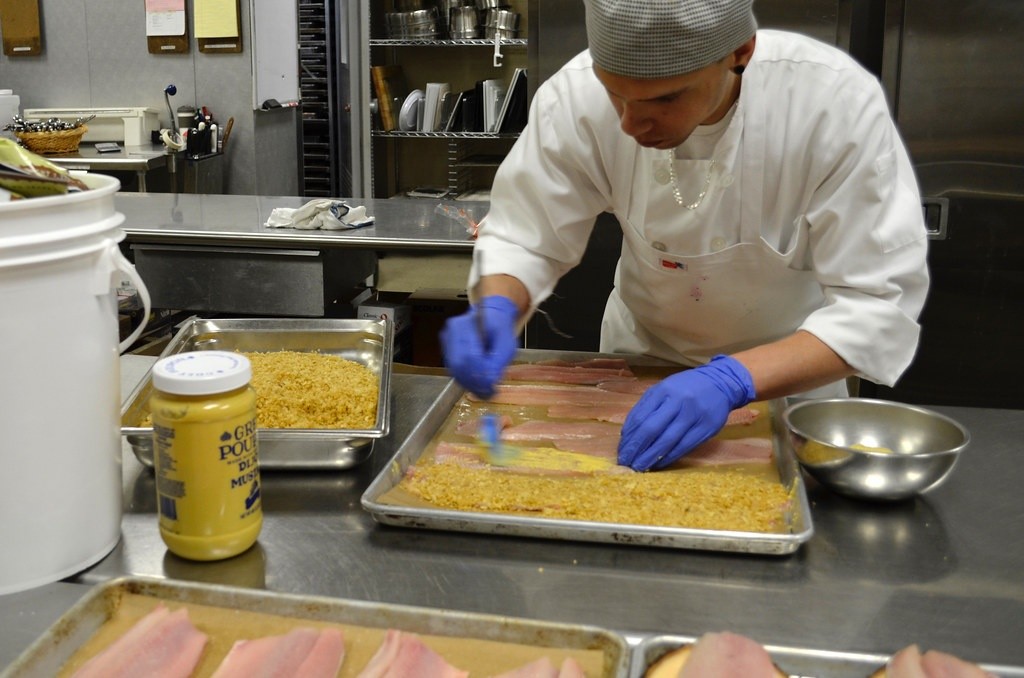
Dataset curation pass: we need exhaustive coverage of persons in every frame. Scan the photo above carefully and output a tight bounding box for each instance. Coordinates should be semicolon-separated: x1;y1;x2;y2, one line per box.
438;0;932;472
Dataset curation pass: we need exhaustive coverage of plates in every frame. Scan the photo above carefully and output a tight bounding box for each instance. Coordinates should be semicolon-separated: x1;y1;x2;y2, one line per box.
399;89;424;131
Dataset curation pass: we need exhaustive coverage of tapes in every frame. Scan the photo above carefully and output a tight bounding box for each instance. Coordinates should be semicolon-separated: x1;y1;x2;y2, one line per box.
163;129;184;148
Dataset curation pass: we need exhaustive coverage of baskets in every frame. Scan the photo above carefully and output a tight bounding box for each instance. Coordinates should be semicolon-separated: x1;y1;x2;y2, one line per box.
15;125;88;153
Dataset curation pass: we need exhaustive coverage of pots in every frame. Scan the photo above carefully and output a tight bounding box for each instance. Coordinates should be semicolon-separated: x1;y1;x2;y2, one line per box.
384;0;523;39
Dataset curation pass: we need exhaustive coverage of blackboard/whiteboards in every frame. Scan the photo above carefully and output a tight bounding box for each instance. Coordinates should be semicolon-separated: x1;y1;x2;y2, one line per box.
249;0;299;112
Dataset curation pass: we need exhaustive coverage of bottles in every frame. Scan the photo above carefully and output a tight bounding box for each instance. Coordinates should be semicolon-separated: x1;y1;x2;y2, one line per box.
0;89;20;143
149;350;262;562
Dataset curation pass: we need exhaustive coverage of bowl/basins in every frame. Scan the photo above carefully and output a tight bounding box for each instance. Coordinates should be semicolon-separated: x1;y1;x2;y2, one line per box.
783;398;971;500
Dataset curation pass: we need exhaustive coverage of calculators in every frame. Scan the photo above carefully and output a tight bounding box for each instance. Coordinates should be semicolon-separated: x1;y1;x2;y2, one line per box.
94;141;121;152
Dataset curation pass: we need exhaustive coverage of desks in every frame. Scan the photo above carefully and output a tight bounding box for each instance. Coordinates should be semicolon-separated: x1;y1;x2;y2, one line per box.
41;143;167;192
0;355;1024;678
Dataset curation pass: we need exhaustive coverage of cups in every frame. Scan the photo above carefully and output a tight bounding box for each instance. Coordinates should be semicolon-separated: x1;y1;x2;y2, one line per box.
190;130;212;154
177;106;195;129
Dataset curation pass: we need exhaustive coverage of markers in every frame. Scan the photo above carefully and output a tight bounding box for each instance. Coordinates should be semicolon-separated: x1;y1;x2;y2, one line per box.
281;102;298;108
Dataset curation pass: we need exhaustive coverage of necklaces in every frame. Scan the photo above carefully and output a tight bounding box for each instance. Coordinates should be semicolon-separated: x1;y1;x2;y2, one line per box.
667;148;716;210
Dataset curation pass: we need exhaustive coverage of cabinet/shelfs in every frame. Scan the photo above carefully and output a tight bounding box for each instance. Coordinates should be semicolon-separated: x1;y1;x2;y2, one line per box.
113;191;534;349
367;0;532;203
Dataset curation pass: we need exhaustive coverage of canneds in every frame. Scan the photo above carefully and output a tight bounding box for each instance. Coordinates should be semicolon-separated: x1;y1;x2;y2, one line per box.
148;351;263;560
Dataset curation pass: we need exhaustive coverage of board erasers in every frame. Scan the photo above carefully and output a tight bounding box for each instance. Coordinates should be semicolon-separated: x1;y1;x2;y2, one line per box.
262;98;282;109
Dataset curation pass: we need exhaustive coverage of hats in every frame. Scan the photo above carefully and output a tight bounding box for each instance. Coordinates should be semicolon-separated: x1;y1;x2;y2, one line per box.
583;0;758;78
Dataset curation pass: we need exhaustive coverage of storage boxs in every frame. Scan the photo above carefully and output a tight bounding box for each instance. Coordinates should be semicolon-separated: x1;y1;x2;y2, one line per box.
359;302;410;335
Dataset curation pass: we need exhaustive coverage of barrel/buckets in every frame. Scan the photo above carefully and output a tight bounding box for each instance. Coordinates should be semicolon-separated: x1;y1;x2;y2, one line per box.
0;173;150;595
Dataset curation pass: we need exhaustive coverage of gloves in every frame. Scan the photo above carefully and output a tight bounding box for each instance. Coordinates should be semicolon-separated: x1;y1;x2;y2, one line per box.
440;296;521;400
616;354;756;472
